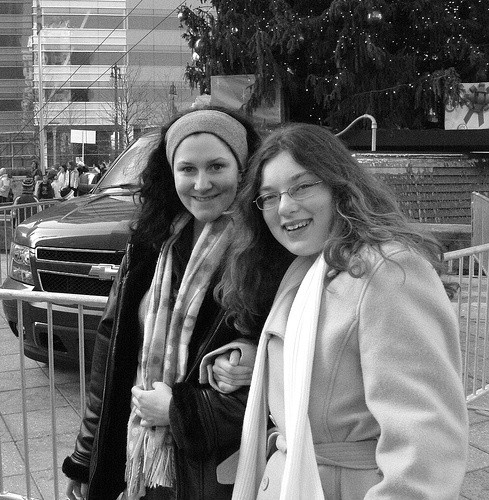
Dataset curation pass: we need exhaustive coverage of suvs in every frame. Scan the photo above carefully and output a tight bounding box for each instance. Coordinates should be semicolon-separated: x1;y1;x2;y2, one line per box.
0;130;163;368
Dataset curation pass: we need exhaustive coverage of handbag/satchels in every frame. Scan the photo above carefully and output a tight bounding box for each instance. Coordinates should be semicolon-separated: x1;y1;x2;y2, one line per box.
60;186;71;197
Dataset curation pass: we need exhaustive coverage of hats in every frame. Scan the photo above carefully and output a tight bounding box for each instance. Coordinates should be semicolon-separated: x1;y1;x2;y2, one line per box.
22;177;34;187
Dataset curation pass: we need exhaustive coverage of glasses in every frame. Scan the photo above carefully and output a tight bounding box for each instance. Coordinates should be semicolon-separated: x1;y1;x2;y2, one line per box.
251;178;324;211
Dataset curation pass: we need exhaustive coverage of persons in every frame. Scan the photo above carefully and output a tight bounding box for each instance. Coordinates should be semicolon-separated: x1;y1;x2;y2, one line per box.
62;105;297;500
0;160;108;228
199;122;470;500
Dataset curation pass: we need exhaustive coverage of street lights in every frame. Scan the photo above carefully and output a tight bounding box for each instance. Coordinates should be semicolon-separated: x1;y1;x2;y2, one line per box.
110;65;122;157
167;83;178;118
